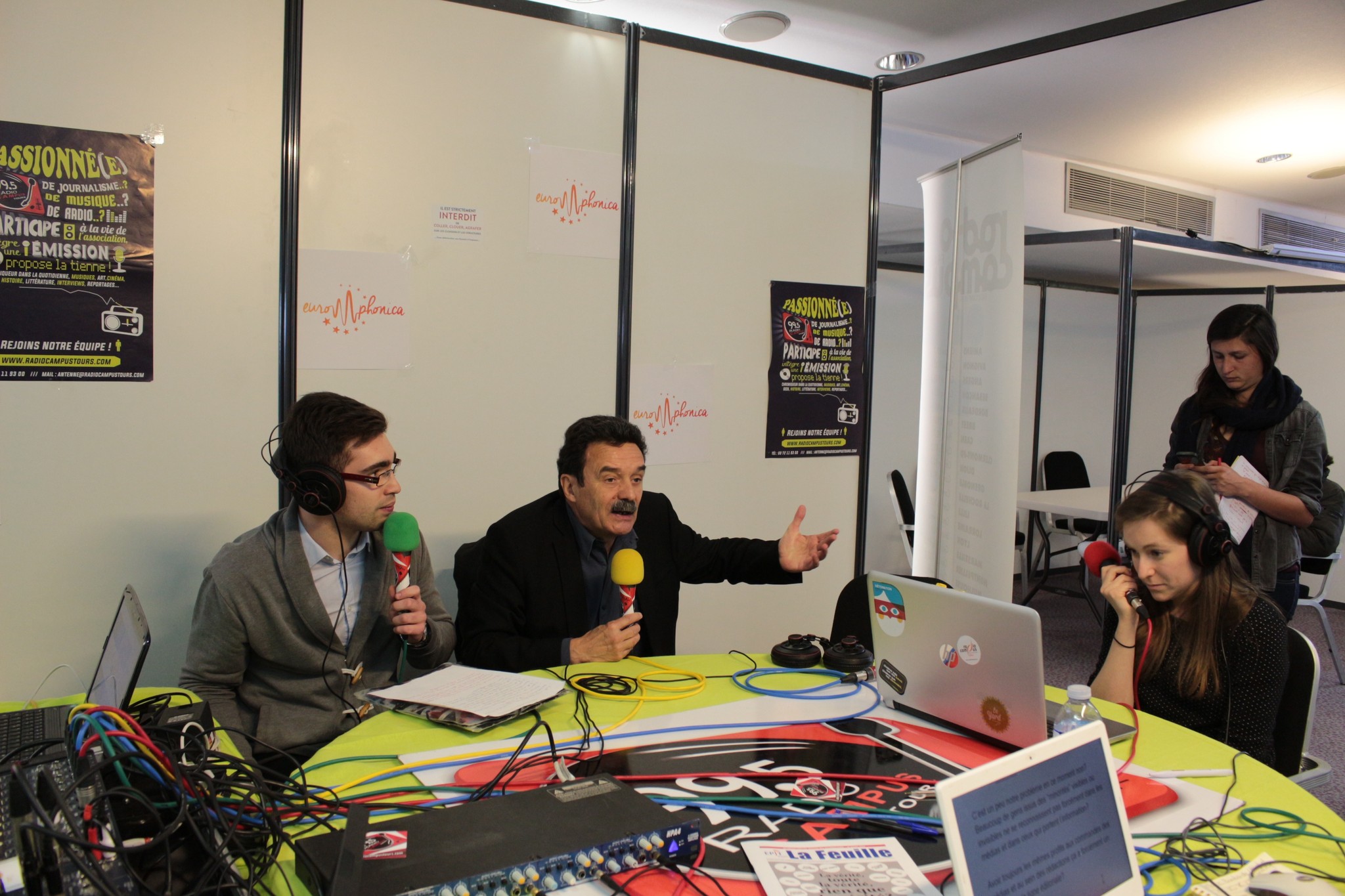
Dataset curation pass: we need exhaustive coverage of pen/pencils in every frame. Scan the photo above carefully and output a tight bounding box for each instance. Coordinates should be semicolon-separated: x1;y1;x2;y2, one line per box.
1217;457;1223;500
1146;768;1232;778
859;818;938;837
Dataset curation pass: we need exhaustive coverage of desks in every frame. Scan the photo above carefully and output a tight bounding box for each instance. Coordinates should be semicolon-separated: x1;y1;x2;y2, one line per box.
0;651;1345;896
1016;484;1137;628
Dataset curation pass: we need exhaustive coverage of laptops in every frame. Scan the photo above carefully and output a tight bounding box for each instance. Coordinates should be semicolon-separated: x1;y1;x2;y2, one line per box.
865;570;1138;755
935;717;1144;896
0;583;152;776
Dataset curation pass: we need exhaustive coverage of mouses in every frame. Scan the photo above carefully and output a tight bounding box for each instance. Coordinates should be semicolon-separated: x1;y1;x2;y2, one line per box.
1248;870;1344;896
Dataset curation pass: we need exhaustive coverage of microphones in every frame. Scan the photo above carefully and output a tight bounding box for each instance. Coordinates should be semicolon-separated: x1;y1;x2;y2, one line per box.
1083;541;1150;622
610;548;645;631
383;511;421;643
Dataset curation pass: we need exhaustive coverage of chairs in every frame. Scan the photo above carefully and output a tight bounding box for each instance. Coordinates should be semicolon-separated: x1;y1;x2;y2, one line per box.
1026;451;1107;593
888;469;1026;571
1281;518;1344;793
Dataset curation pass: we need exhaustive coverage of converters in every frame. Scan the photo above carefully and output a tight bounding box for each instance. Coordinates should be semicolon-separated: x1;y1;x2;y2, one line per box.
154;700;213;752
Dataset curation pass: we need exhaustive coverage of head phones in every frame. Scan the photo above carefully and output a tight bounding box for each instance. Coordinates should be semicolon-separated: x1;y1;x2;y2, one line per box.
1124;467;1235;573
769;630;873;672
261;420;346;517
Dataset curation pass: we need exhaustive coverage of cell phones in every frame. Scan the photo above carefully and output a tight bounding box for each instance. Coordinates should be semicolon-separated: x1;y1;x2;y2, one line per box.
1174;451;1208;472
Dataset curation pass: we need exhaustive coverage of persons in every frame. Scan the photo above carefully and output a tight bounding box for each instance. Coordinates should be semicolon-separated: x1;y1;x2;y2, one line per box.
1086;304;1332;778
453;416;840;673
1086;470;1286;775
179;392;839;791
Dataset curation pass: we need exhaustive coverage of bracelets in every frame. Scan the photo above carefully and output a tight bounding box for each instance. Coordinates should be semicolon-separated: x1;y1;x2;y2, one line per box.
1113;636;1136;648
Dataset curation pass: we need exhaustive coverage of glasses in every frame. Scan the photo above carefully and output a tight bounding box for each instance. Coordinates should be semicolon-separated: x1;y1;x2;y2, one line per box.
340;458;402;487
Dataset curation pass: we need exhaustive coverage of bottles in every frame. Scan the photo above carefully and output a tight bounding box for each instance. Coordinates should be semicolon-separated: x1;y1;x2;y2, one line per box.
1052;684;1103;738
